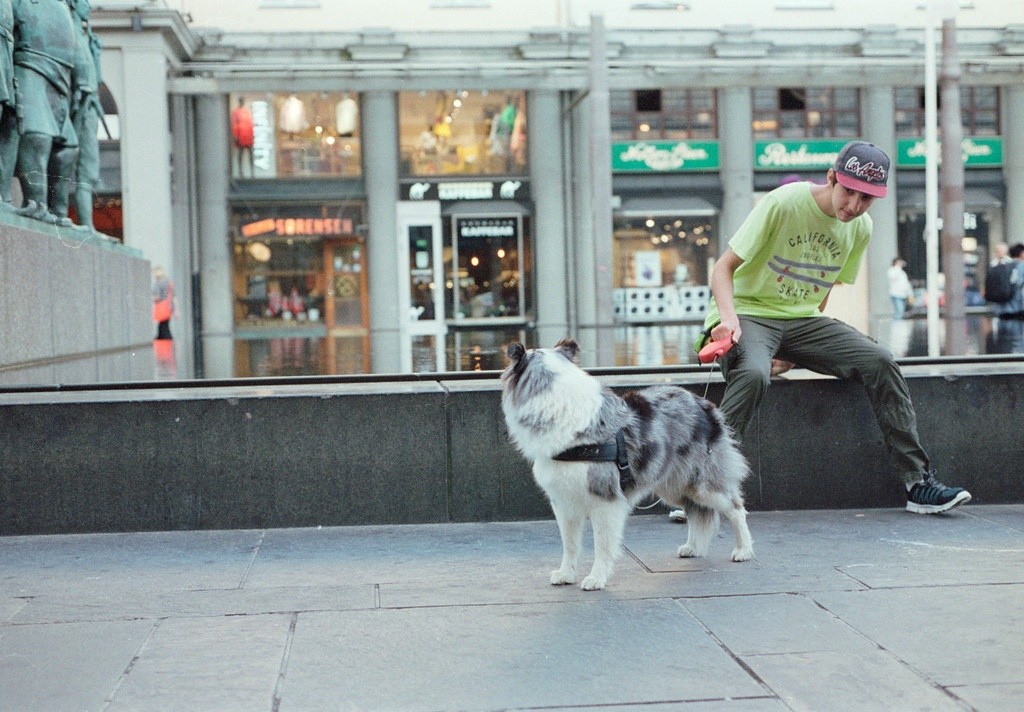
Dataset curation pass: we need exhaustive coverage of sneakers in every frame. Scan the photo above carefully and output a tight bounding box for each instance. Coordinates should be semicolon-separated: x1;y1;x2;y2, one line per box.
904;467;972;515
668;507;688;522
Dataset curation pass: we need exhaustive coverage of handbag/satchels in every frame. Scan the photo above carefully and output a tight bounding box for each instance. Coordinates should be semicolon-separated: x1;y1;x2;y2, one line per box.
903;295;915;312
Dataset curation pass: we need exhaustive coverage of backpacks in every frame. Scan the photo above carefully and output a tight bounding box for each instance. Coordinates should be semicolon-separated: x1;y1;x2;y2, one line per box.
983;260;1018;305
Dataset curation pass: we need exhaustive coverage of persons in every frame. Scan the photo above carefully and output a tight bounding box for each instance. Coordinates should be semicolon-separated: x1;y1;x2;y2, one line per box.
983;241;1023;358
418;98;526;173
228;96;360;177
1;2;101;235
151;264;176;342
886;257;914;321
670;142;973;521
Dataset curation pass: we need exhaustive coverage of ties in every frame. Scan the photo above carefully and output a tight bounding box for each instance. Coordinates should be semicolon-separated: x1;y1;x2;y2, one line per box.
997;258;1002;265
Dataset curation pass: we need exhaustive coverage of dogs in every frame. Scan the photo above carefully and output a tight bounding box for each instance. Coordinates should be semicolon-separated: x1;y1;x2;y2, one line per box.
499;337;755;591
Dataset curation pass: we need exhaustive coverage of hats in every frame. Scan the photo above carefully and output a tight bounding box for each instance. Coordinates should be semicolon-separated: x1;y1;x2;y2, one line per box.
833;141;891;198
